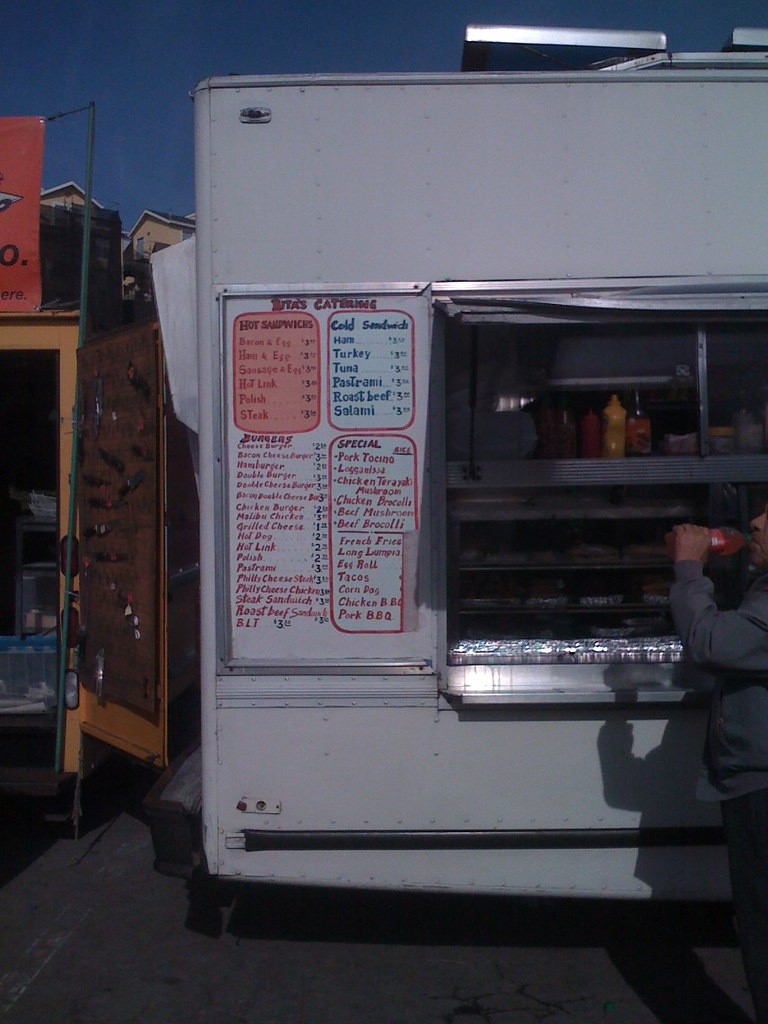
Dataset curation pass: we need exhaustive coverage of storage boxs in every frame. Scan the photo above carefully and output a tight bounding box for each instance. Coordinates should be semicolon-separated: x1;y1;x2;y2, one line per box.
0;635;59;711
22;570;58;634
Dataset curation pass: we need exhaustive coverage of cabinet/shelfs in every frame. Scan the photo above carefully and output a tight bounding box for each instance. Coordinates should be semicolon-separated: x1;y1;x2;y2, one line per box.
445;452;768;642
14;514;58;638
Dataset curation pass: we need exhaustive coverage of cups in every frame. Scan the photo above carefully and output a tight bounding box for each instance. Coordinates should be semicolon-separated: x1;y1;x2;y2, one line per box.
554;424;576;458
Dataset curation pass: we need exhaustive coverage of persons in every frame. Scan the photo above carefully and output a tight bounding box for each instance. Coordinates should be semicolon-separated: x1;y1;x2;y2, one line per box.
672;509;768;1024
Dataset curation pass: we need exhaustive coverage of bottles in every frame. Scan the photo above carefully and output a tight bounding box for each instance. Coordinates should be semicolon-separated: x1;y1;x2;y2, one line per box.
666;527;751;561
625;385;652;456
735;391;752;449
582;409;600;457
602;395;627;457
